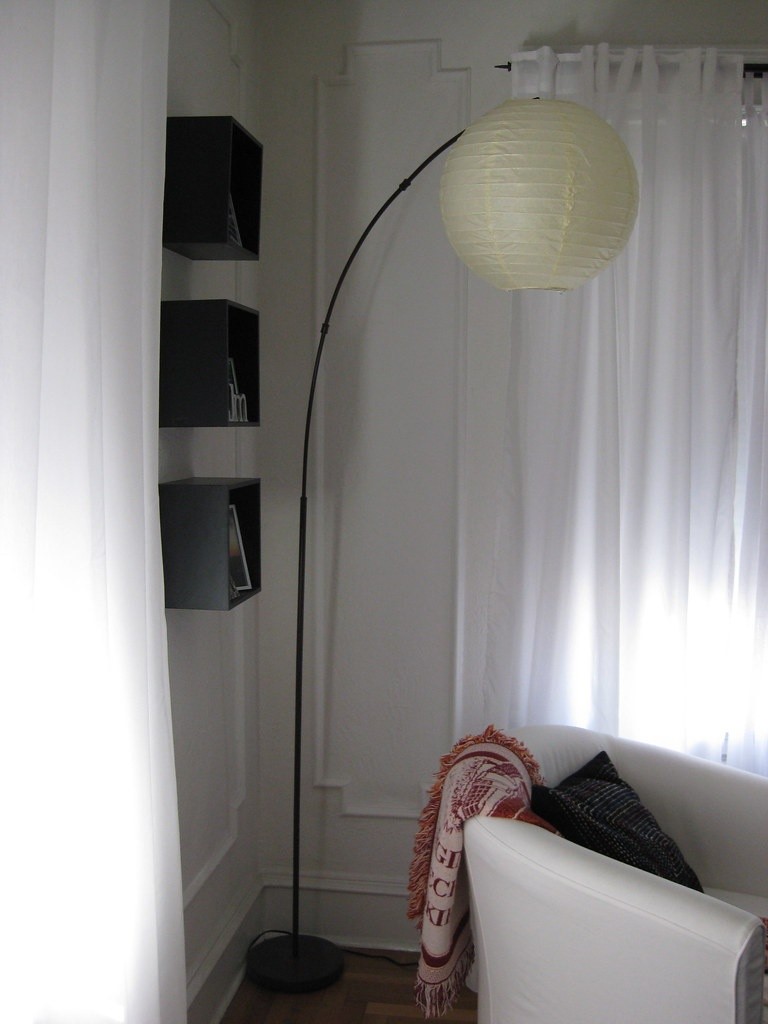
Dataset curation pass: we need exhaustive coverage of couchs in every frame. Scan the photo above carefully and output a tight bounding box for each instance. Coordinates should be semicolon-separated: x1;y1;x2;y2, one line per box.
462;724;767;1024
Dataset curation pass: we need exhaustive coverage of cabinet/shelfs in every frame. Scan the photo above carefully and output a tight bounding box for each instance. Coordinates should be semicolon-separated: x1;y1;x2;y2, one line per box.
159;113;262;611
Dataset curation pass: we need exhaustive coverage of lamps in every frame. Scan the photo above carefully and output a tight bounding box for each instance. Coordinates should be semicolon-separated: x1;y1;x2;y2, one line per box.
240;94;638;996
